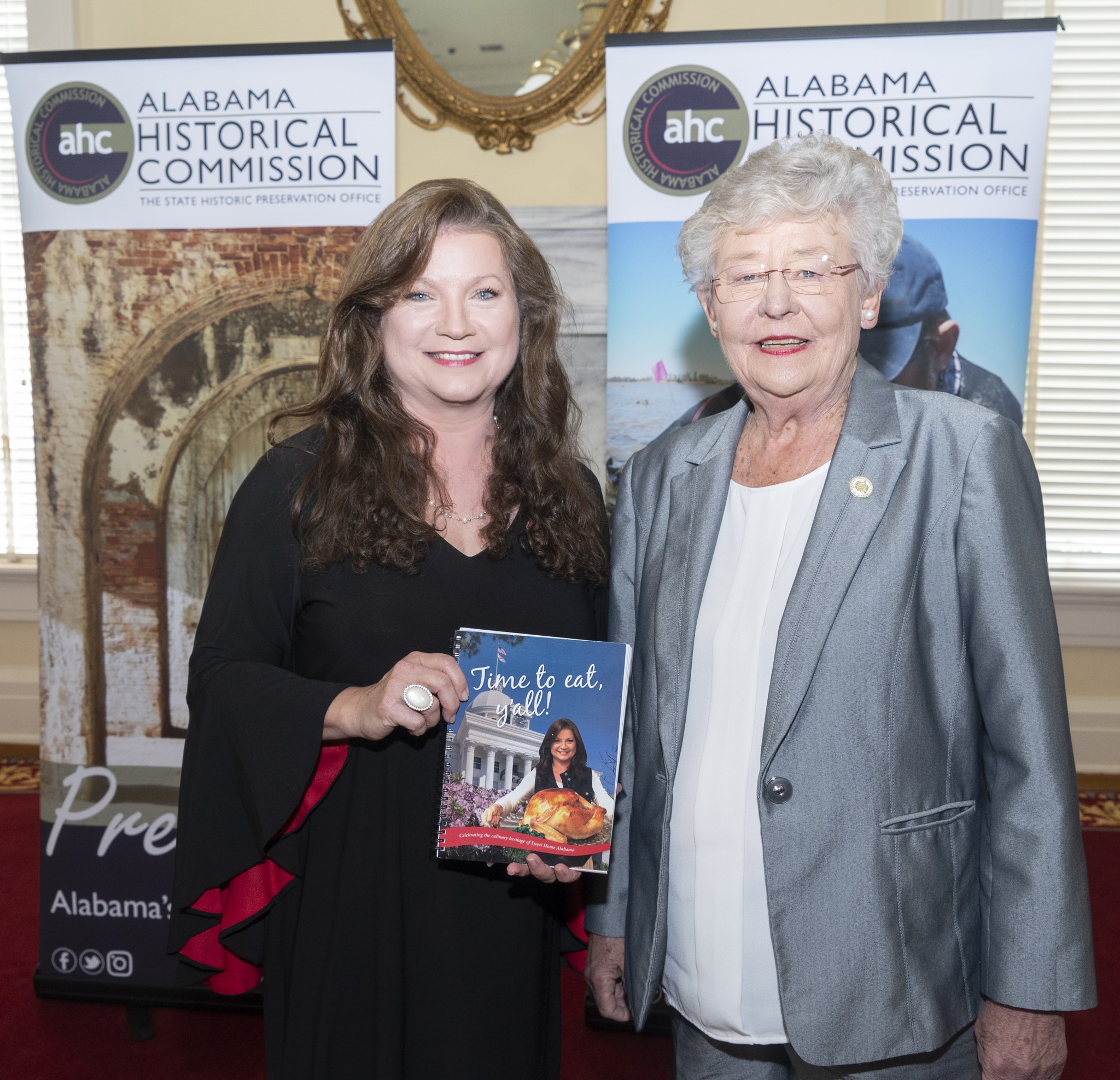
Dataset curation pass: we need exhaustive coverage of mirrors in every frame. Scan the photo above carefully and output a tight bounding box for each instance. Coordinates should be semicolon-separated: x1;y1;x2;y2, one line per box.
335;0;673;154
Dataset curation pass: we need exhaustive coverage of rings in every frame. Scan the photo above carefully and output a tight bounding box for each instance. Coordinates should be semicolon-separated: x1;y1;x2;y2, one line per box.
403;684;433;711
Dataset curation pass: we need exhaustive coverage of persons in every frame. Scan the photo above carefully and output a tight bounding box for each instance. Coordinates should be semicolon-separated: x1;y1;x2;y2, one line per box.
857;234;1023;433
480;718;614;828
165;178;614;1080
582;128;1098;1080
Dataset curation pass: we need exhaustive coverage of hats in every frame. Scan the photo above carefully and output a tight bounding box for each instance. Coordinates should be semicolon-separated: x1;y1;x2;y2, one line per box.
856;233;948;384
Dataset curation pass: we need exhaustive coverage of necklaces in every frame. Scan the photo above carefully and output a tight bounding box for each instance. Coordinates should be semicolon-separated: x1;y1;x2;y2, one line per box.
746;407;846;488
427;496;488;524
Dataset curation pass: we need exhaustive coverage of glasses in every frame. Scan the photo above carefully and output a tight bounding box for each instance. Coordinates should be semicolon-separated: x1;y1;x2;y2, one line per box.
714;257;863;308
